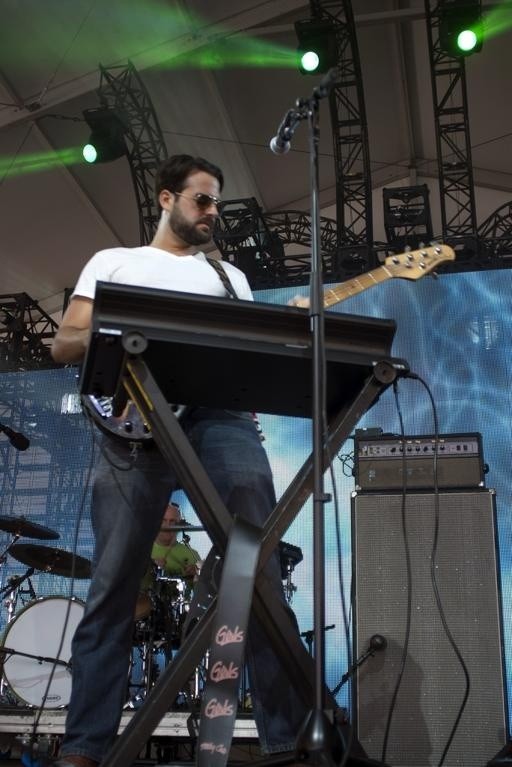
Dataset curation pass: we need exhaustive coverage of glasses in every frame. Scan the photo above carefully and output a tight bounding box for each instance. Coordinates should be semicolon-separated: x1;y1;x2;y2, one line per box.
175;191;224;213
169;501;179;509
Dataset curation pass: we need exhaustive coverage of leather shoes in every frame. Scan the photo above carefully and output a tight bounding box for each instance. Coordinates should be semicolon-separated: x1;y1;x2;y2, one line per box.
48;755;99;767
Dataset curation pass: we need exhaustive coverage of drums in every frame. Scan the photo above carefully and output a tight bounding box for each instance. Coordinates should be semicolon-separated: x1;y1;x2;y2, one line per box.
132;579;200;650
1;594;132;710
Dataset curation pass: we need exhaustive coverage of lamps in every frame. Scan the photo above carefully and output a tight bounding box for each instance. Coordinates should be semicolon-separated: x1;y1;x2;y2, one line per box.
290;6;486;76
81;105;132;165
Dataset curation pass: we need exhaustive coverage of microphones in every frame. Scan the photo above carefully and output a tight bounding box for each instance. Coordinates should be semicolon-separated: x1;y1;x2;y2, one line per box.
28;579;37;601
270;108;296;155
0;421;30;451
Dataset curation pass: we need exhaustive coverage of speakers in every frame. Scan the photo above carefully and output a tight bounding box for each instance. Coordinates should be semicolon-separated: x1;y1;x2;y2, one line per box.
350;486;509;767
356;431;484;492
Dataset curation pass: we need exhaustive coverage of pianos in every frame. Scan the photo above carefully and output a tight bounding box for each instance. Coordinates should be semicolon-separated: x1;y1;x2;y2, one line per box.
80;280;394;422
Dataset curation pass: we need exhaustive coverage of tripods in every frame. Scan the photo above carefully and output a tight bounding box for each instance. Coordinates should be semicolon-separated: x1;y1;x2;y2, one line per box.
120;583;208;706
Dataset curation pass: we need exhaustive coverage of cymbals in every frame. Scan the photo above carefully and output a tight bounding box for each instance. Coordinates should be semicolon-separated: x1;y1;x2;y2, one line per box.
0;513;60;539
9;543;94;579
162;525;205;531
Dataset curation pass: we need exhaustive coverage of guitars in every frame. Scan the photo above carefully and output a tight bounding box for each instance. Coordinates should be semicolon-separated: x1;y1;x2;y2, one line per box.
79;245;459;441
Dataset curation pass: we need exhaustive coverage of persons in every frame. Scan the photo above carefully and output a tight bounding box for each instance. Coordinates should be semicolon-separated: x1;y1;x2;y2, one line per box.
124;503;202;708
50;152;314;767
